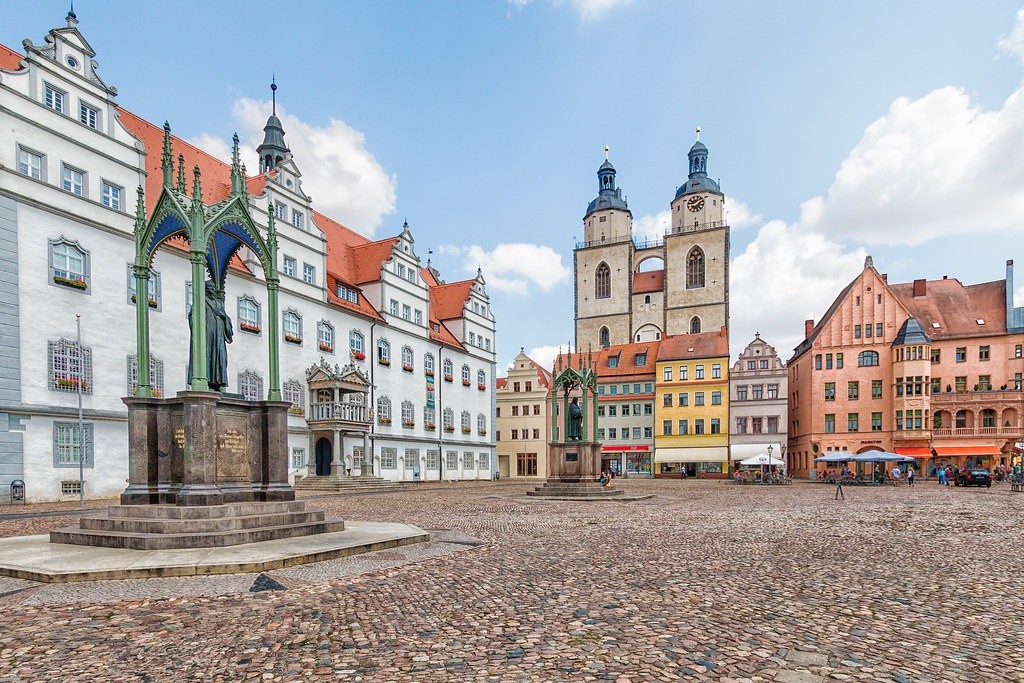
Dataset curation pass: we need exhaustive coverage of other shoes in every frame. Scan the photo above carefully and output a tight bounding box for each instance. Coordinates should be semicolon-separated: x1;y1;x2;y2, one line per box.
912;483;914;487
945;484;948;486
908;485;910;486
894;485;895;487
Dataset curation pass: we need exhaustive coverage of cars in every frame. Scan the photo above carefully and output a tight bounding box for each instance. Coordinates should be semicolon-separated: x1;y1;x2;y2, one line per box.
954;468;992;487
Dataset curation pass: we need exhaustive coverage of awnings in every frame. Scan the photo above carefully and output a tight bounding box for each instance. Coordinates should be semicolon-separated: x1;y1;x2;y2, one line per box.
894;448;933;459
935;445;1003;457
654;449;728;462
731;444;782;461
601;450;653;453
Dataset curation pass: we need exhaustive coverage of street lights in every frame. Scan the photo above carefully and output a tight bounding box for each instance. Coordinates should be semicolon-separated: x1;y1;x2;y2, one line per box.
767;445;774;485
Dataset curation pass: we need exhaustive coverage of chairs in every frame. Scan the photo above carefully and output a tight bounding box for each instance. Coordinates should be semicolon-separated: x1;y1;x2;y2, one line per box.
742;473;794;485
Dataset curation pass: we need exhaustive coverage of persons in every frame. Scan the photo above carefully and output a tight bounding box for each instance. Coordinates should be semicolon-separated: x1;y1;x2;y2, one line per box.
187;280;233;392
611;466;616;479
938;465;1024;492
568;397;583;440
872;463;890;483
601;470;610;485
891;466;901;487
819;466;865;483
907;465;915;487
681;464;687;479
732;467;784;484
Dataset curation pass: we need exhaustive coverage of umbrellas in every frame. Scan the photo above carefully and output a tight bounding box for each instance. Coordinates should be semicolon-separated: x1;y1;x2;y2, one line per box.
849;449;904;484
740;453;785;484
882;451;915;474
815;452;855;470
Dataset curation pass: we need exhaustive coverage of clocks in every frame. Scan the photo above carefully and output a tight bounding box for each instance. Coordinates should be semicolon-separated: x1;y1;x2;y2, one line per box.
687;195;705;213
